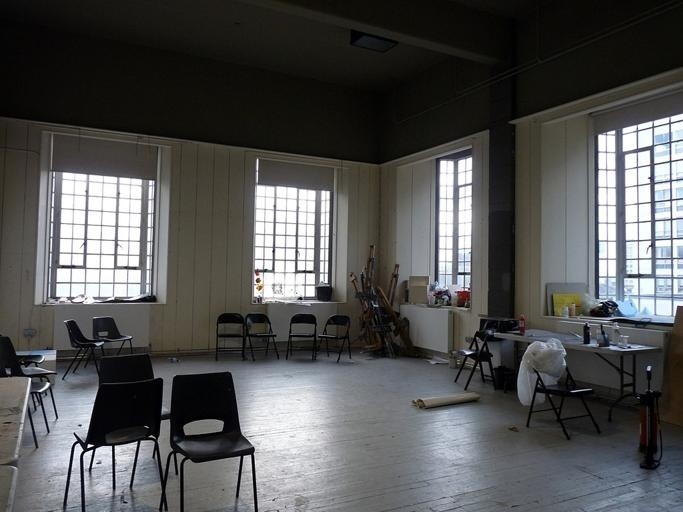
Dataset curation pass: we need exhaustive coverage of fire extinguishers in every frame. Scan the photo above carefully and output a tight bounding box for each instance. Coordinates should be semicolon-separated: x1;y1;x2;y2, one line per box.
638;397;658;454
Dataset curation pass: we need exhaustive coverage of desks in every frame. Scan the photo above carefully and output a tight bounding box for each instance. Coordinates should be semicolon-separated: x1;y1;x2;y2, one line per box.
490;328;664;427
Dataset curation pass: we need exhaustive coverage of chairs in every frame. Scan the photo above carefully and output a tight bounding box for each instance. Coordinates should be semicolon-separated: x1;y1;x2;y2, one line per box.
241;313;279;361
524;362;603;440
316;313;352;363
453;328;497;393
283;313;317;361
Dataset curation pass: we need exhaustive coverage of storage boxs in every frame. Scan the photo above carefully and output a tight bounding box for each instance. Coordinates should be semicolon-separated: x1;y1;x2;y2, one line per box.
408;283;429;305
406;274;430;288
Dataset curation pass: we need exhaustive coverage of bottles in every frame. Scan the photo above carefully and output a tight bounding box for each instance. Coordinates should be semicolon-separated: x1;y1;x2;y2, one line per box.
562;301;575;317
428;281;470;307
519;314;525;336
583;323;590;342
611;322;619;344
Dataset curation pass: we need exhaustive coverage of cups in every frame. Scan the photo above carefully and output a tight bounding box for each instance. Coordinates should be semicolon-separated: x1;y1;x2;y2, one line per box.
619;335;629;346
597;328;609;347
